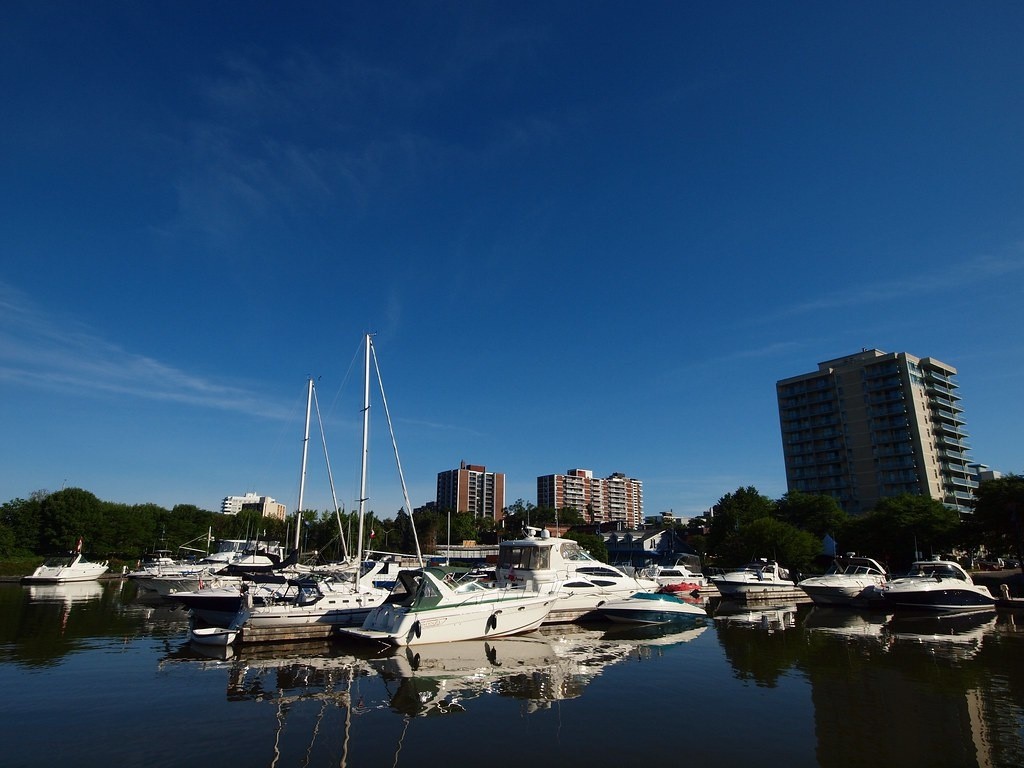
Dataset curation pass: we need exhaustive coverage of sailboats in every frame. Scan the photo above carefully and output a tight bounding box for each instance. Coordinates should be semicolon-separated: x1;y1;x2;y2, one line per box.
169;330;427;623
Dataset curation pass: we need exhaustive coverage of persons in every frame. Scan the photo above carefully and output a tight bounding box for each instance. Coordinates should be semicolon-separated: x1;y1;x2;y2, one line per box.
506;562;516;581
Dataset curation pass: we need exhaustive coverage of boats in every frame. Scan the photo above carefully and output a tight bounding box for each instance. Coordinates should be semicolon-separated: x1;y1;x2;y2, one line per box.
22;581;107;618
339;565;571;647
125;523;296;595
365;633;582;716
880;561;996;607
21;552;109;582
708;546;815;607
797;552;887;611
191;628;238;646
880;612;999;661
803;606;898;641
710;601;798;634
637;566;710;590
600;623;708;645
596;591;707;624
